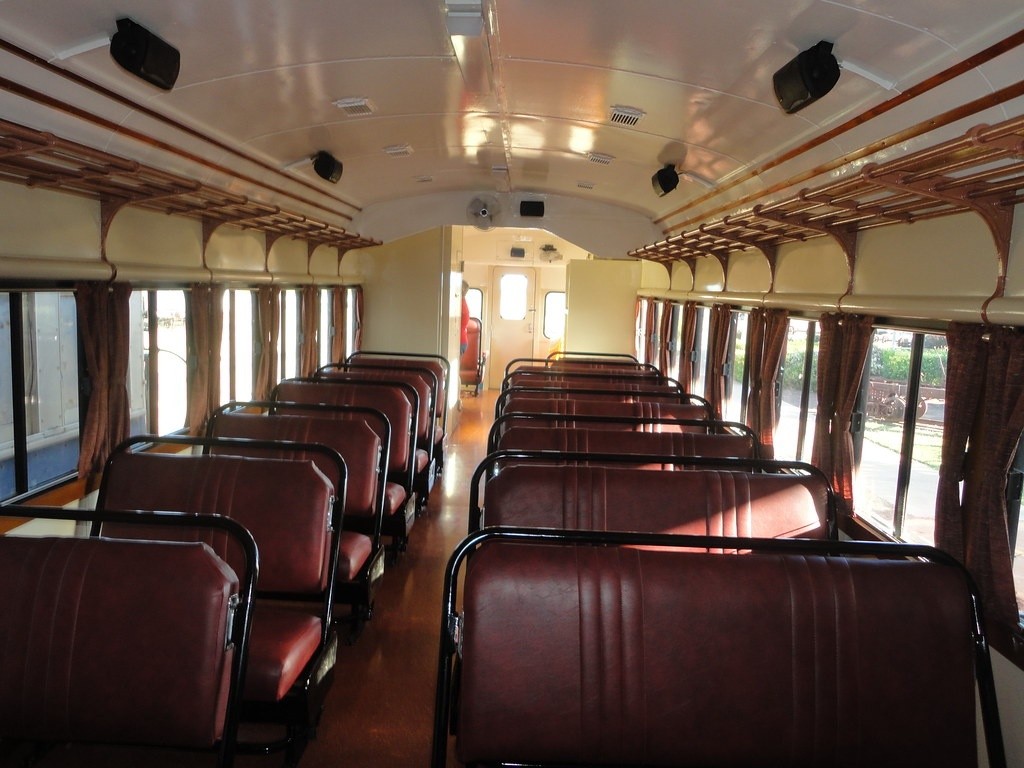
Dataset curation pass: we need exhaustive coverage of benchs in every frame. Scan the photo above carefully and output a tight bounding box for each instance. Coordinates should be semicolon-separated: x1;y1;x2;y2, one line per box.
0;318;1005;768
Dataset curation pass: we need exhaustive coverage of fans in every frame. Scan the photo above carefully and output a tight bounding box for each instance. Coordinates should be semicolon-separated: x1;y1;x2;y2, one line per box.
466;193;503;232
